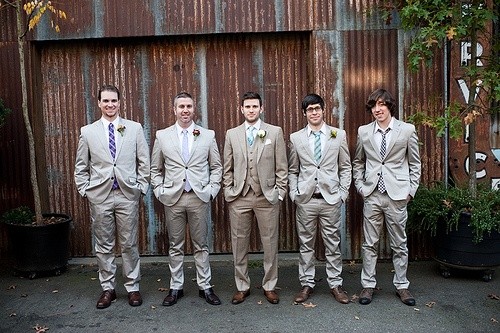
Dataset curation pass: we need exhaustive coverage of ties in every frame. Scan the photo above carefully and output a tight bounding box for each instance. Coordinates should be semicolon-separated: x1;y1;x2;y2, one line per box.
378;128;390;194
248;126;255;146
182;130;191;192
313;131;322;166
108;123;118;190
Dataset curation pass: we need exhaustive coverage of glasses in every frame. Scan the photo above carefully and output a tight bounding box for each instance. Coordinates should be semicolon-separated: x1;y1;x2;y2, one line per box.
304;106;322;112
371;101;387;108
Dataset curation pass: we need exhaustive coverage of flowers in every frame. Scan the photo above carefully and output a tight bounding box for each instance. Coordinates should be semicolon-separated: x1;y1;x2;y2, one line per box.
193;128;200;141
117;124;126;137
258;130;266;142
328;129;337;141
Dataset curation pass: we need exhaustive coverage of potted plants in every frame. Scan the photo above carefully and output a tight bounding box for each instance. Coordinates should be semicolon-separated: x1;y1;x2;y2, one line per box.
363;0;500;281
0;0;73;277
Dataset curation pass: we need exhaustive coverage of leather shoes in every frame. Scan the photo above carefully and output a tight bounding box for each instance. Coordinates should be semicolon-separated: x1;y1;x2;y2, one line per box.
127;292;144;307
329;286;351;303
96;290;117;309
198;289;220;305
231;290;251;304
295;285;315;303
264;290;279;304
162;288;184;307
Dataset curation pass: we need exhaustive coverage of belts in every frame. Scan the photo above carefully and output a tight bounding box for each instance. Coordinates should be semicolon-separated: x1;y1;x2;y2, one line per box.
312;193;324;199
376;185;378;190
183;188;193;193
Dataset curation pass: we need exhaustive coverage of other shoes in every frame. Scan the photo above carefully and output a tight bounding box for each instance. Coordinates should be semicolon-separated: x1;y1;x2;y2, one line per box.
395;288;416;305
358;288;374;304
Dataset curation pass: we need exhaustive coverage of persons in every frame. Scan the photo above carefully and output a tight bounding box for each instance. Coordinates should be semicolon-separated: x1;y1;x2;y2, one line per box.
287;94;352;304
352;88;421;306
74;86;151;309
222;92;289;305
148;92;223;305
